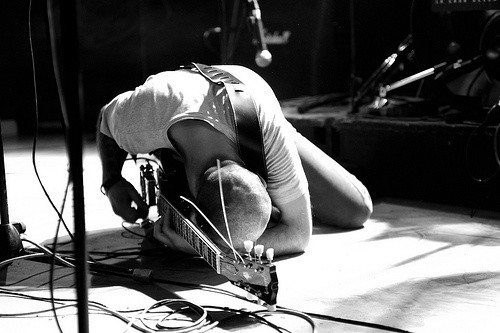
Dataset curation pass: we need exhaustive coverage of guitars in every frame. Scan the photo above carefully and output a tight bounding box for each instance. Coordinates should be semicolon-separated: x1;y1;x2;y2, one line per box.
139;165;277;312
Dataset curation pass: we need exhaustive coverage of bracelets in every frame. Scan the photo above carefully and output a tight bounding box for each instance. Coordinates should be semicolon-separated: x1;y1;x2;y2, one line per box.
100;172;123;195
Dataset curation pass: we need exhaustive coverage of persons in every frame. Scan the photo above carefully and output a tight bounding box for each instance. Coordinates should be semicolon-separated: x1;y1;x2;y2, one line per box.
96;62;375;275
